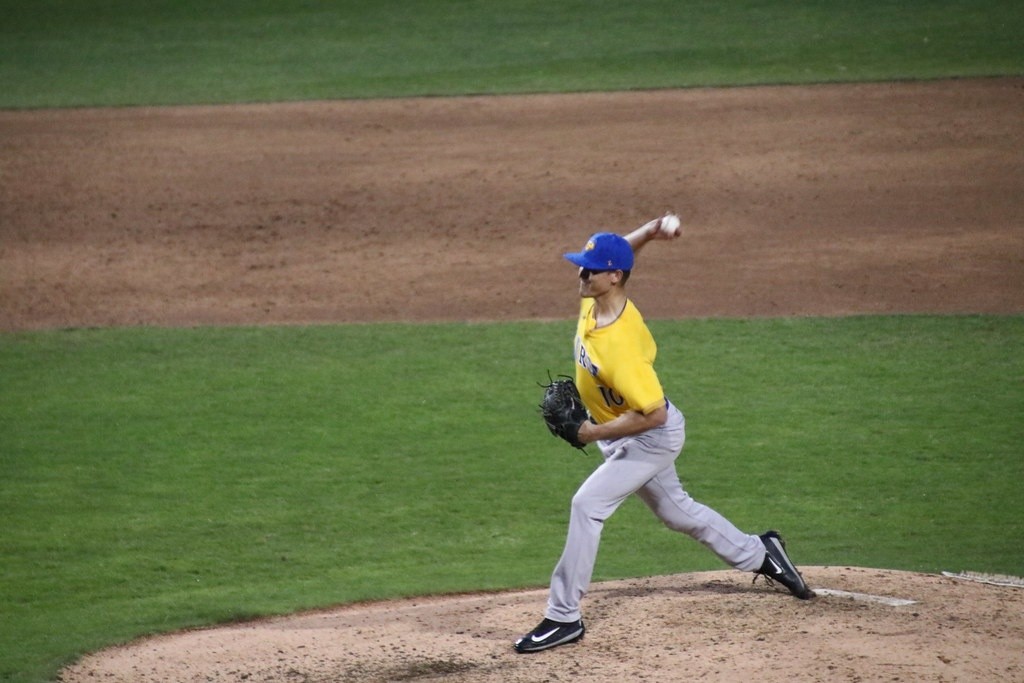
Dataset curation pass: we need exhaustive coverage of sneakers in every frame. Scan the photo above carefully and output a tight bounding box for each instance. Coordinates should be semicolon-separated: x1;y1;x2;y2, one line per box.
514;618;585;652
752;530;810;600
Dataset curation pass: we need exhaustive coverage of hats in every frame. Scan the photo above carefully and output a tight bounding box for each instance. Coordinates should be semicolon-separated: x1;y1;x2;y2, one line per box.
563;233;634;270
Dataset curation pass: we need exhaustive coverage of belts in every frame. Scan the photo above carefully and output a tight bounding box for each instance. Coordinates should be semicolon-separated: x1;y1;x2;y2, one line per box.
664;397;669;409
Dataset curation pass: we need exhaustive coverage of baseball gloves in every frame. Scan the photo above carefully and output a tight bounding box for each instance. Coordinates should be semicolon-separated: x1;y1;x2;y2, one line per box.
535;369;598;457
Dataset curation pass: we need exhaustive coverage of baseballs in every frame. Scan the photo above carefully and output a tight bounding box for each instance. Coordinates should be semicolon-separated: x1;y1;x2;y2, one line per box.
660;215;681;235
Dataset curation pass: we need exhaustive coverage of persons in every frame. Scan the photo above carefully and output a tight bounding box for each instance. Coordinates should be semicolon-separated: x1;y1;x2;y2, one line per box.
513;210;810;653
